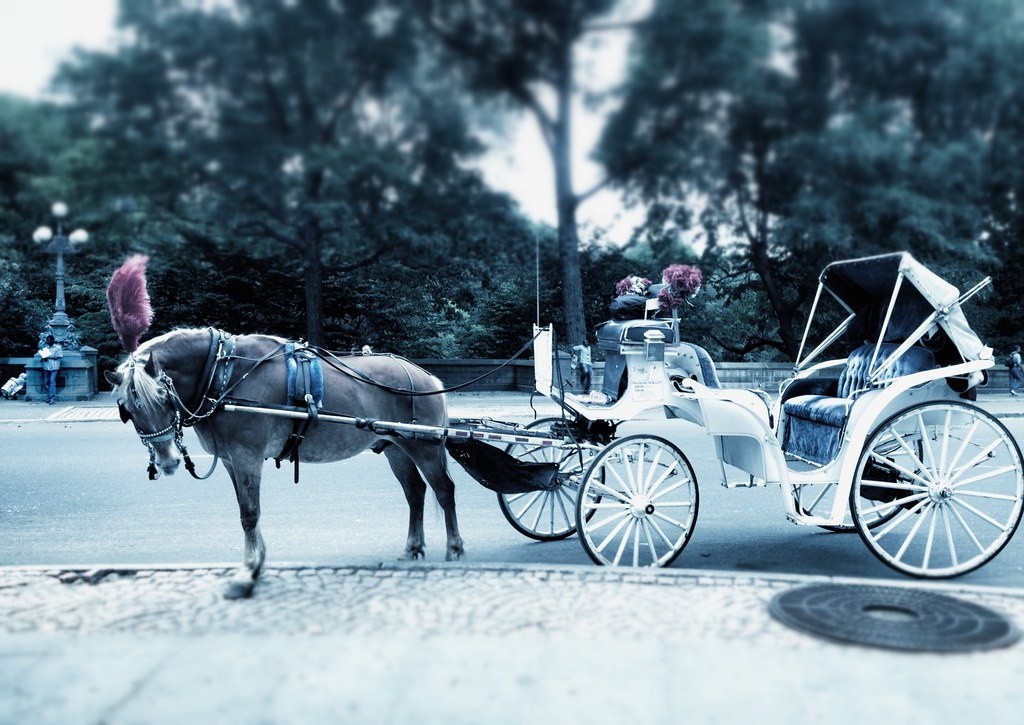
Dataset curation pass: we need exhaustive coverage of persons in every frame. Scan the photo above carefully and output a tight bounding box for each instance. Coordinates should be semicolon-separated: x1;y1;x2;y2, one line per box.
571;339;593;394
350;346;359;355
1008;345;1024;396
40;335;63;405
363;345;372;354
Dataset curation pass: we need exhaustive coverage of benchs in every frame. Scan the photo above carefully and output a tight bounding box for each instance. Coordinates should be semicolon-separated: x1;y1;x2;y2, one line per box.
594;283;681;355
781;343;935;429
664;342;774;428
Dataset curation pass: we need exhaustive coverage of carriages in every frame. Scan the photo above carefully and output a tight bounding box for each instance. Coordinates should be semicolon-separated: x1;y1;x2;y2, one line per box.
100;248;1024;602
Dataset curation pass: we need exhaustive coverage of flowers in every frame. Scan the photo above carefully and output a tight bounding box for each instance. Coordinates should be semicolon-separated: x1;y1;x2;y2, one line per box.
659;264;703;312
616;275;653;296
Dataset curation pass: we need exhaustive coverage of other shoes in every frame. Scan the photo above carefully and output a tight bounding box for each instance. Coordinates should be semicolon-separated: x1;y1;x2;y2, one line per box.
45;397;55;405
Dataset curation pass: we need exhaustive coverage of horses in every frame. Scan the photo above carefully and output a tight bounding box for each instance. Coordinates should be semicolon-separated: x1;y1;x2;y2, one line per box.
103;324;465;601
